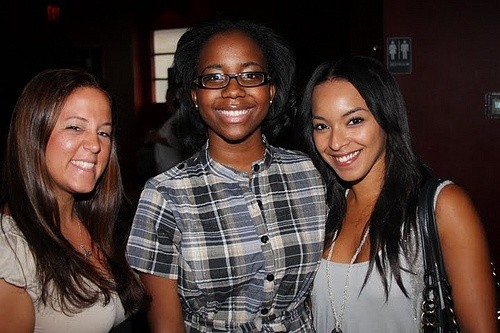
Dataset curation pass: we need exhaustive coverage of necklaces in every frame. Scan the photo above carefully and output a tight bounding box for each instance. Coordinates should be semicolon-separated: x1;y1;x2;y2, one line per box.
326;188;370;333
61;216;92;258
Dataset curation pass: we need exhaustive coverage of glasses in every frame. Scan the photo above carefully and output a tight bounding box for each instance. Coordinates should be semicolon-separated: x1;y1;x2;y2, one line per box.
194;71;272;90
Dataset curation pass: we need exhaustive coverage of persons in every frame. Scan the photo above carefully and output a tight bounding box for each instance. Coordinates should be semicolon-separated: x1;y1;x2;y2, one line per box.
0;69;153;333
301;57;497;333
124;22;348;333
